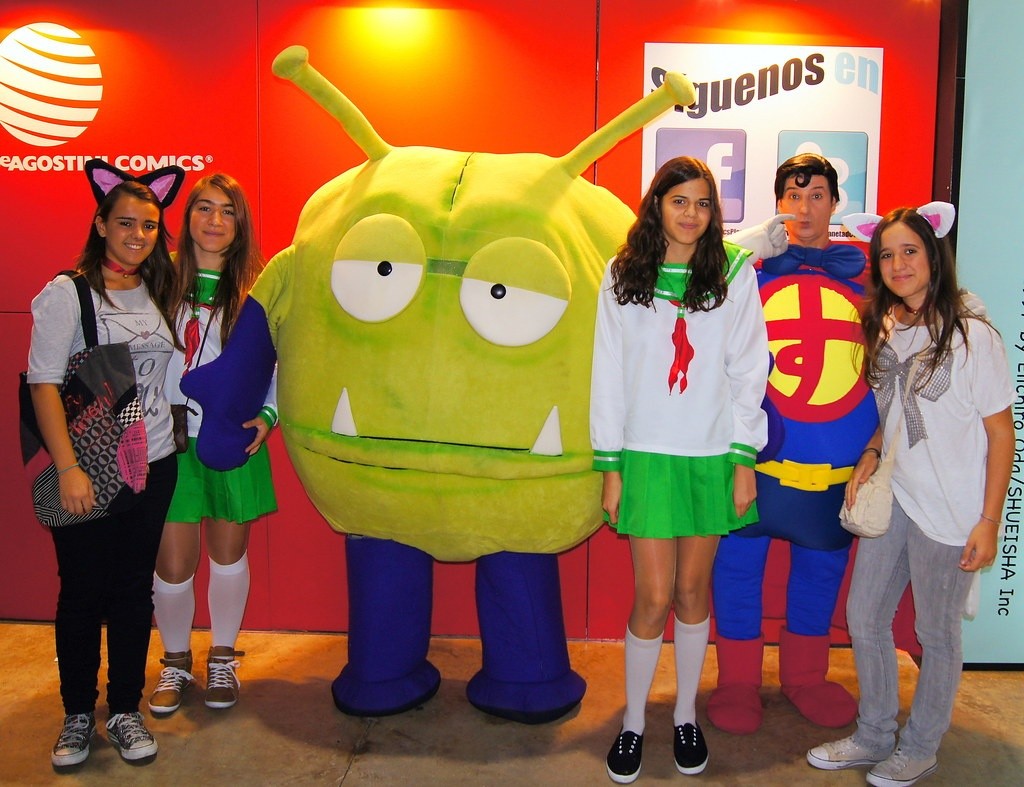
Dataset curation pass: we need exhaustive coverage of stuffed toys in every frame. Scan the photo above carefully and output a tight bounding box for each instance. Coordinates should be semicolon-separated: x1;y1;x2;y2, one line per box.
178;44;697;722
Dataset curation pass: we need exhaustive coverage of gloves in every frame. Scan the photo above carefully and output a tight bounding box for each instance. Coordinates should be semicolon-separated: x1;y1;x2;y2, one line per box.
961;289;994;322
723;213;797;266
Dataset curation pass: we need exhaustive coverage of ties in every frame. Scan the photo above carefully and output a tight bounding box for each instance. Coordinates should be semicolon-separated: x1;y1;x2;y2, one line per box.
666;299;695;396
183;303;215;371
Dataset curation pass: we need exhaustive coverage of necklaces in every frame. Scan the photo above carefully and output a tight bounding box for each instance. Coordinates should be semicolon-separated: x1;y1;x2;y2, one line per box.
905;305;924;315
104;257;138;278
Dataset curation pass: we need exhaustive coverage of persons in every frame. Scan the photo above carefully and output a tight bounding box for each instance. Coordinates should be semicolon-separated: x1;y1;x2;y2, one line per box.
589;156;771;785
806;208;1016;787
708;153;988;736
149;173;279;713
26;178;186;767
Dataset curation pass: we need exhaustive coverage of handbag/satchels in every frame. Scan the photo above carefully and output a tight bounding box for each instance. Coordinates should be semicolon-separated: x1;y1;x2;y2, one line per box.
168;403;191;454
963;569;981;619
839;457;895;538
18;268;149;528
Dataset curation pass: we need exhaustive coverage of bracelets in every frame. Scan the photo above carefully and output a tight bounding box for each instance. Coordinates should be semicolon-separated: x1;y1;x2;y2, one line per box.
57;462;78;473
982;514;1001;524
863;448;880;457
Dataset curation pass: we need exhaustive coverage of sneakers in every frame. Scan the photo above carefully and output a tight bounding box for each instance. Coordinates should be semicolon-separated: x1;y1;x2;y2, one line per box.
865;744;939;787
605;723;644;784
806;734;886;770
204;645;246;709
148;648;197;714
105;711;159;761
50;712;97;766
672;720;709;775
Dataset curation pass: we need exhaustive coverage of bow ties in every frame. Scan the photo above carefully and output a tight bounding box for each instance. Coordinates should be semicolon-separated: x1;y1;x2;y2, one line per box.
760;241;867;280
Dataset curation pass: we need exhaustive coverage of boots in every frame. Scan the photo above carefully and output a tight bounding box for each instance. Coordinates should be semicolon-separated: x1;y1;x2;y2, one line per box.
708;633;764;736
778;625;858;729
